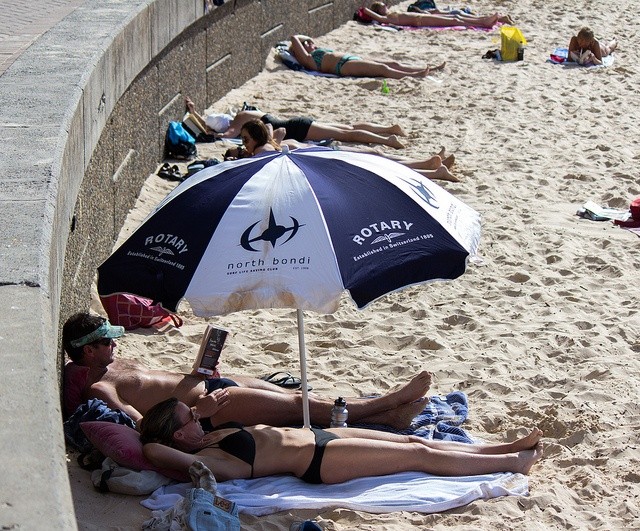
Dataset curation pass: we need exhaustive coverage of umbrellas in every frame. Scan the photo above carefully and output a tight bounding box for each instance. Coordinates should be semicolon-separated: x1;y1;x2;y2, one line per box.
97;145;481;320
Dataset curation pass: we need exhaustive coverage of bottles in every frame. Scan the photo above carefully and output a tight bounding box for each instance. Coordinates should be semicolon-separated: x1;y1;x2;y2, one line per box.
518;41;524;60
330;397;348;428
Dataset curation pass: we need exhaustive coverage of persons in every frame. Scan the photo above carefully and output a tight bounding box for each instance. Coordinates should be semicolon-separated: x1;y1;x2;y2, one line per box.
63;312;432;432
567;27;619;64
240;118;460;187
364;2;498;29
142;396;544;484
407;6;515;25
222;147;455;198
289;34;446;79
185;95;409;150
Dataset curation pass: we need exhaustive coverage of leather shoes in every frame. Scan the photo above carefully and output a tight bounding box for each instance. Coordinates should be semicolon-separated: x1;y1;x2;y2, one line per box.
170;165;180;181
157;162;171;178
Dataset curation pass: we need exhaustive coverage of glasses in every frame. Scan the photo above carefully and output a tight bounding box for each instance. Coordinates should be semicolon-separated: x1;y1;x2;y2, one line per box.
85;338;111;346
240;136;251;145
172;406;201;438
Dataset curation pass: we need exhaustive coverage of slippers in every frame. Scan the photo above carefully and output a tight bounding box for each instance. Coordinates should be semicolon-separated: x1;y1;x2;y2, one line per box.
254;371;312;392
289;519;325;531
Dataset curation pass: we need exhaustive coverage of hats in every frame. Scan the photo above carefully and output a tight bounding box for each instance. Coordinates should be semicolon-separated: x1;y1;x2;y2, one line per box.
70;319;125;349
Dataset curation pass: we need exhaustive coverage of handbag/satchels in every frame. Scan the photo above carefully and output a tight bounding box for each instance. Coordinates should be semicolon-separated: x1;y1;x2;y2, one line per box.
101;294;169;330
501;26;527;61
166;121;197;160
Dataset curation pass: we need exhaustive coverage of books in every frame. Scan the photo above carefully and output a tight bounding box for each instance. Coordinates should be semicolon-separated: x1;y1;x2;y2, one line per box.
579;48;591;64
183;112;210;137
575;199;631;221
192;323;229;376
